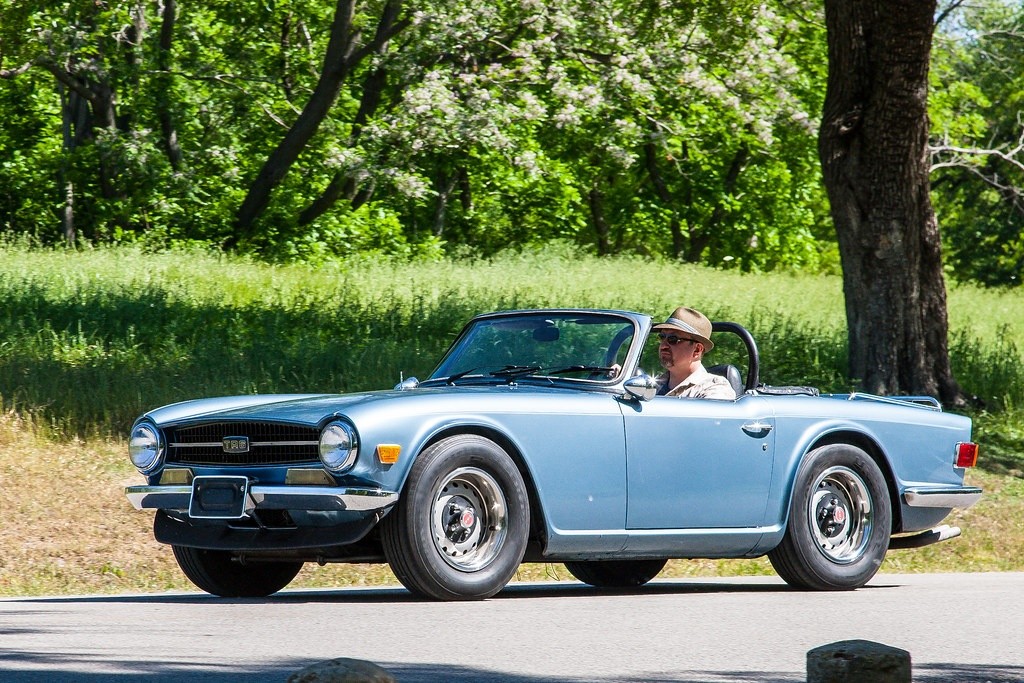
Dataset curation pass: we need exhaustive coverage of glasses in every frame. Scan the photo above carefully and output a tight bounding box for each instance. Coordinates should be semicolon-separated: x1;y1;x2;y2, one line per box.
657;333;700;345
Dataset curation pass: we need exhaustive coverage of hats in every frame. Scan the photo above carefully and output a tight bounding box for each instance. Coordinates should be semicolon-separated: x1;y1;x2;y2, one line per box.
653;307;714;353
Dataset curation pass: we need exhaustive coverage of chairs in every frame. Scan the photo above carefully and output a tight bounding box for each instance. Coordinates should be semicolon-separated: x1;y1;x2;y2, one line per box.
707;364;743;397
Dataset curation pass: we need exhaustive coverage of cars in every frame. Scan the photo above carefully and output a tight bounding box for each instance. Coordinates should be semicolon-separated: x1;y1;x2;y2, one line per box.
124;309;983;602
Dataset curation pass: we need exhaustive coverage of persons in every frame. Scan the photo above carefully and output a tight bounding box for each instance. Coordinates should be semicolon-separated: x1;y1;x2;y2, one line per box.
609;307;737;400
